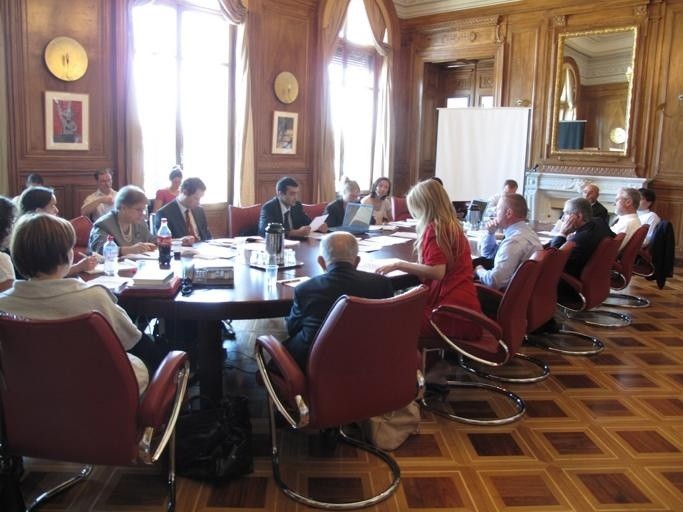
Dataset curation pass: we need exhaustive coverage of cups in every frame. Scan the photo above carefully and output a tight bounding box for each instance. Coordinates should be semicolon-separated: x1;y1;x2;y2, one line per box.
265;264;279;285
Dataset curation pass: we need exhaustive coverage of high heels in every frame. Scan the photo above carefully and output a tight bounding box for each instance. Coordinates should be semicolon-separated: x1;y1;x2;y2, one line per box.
425;382;450;402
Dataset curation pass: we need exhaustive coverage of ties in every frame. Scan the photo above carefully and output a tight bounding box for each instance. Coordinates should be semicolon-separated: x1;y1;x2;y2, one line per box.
609;218;619;227
184;210;198;242
284;211;290;232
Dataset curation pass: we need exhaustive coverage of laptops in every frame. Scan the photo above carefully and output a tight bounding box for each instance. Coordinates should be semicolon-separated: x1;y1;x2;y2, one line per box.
327;202;374;235
452;201;471;218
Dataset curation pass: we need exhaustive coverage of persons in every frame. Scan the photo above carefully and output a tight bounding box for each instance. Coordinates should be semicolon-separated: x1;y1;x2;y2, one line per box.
2;212;157;395
325;175;377;228
481;179;519;224
634;187;663;269
1;172;98;289
542;197;618;306
375;176;481;395
360;177;394;224
552;183;607;241
259;176;328;239
609;186;642;283
81;164;211;253
475;191;543;322
273;230;393;376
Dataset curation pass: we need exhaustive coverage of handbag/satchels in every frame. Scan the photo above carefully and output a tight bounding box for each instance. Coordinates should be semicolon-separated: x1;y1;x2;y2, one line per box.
149;393;253;484
370;400;421;450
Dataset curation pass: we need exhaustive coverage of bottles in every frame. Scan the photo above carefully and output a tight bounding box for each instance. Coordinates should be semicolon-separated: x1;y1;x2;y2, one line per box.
156;217;173;267
102;235;118;277
467;204;481;225
264;223;286;267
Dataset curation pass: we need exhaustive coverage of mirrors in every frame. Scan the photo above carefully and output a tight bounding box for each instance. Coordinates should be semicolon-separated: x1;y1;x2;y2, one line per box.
550;25;642;159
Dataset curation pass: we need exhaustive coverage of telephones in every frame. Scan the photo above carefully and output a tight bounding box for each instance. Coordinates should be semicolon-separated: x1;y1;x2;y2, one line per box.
179;264;208;296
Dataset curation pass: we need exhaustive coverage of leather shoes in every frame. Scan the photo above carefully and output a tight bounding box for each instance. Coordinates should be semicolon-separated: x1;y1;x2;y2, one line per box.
222;320;235;337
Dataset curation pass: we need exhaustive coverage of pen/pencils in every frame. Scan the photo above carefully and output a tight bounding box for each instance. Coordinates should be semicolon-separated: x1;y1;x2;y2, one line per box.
281;279;301;284
78;251;87;257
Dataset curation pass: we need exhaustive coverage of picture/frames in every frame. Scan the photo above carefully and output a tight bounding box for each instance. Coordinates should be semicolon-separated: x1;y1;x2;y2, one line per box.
271;110;299;154
45;90;89;151
